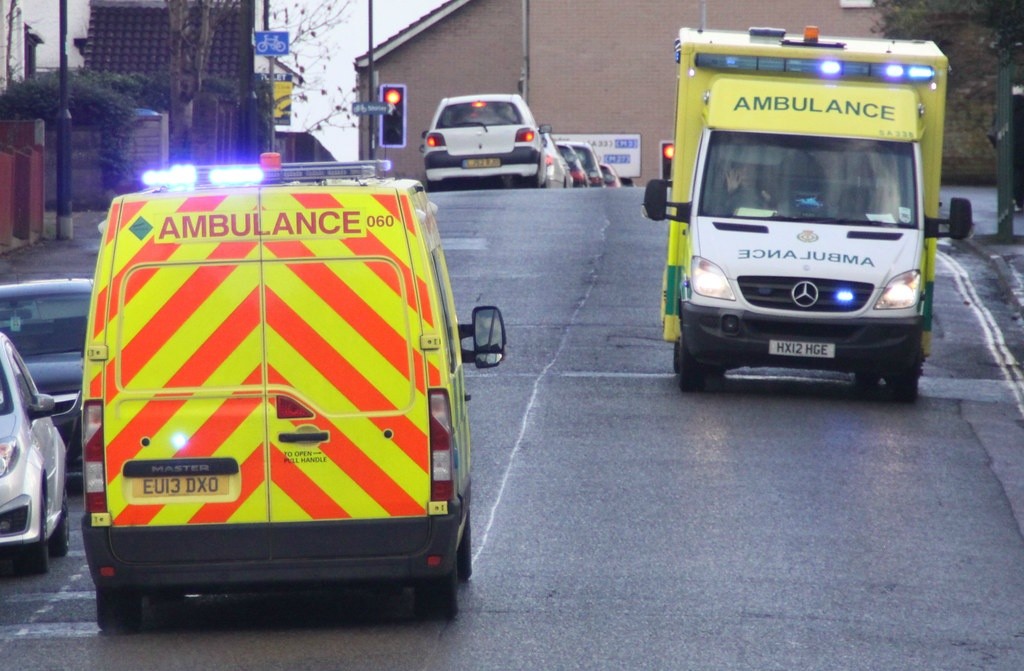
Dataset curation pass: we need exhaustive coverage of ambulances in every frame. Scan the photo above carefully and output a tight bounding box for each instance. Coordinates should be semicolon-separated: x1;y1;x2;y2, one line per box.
641;27;975;397
82;153;509;632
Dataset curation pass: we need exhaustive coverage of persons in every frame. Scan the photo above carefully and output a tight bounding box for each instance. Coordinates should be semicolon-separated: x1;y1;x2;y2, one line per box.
718;155;777;215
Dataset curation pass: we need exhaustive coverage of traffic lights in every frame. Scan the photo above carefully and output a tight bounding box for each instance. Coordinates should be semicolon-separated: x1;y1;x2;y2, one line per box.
659;140;675;182
378;84;407;147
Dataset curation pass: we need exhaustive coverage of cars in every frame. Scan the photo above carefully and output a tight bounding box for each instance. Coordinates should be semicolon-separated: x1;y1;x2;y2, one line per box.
421;92;552;191
0;279;102;473
0;332;70;576
538;127;633;189
